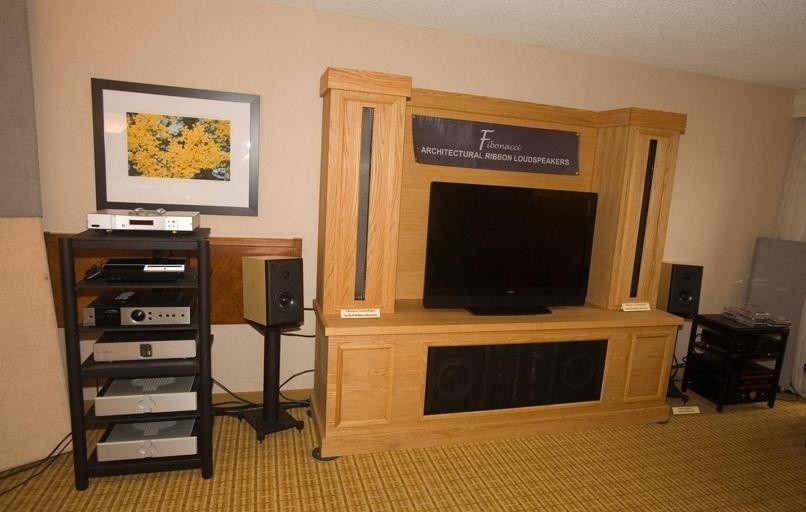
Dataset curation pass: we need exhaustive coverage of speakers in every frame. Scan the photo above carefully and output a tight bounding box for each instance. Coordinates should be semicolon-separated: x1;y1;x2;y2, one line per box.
242;255;304;327
422;338;608;416
656;261;703;320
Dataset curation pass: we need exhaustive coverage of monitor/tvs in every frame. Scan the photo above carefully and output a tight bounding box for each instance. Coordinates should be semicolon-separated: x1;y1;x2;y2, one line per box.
423;181;598;316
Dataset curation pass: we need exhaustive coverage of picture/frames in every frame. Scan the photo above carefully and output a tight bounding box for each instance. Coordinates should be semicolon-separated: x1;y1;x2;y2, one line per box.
90;76;261;216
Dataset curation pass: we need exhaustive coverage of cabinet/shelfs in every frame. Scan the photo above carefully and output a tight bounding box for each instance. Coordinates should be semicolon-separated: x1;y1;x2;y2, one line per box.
681;310;791;415
299;64;686;466
53;221;224;492
